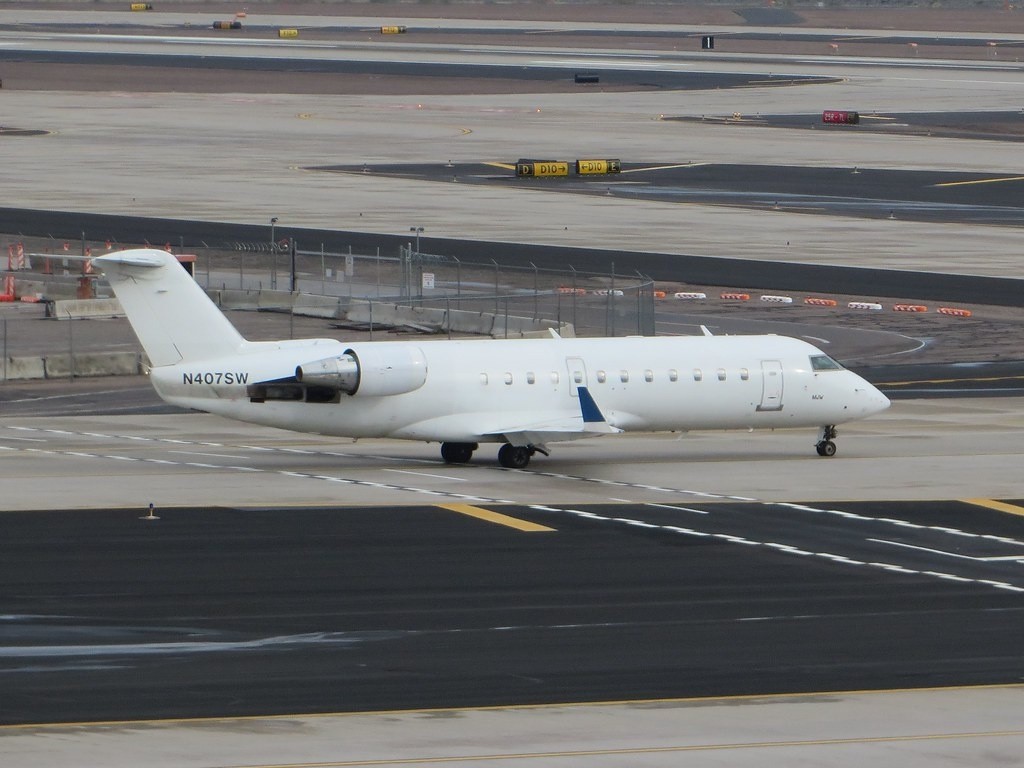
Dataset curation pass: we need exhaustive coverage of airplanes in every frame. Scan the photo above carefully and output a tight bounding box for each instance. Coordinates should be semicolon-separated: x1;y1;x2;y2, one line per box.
24;249;890;469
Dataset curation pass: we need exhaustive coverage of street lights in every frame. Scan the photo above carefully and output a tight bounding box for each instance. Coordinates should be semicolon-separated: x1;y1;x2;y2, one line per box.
270;218;279;290
410;227;425;298
